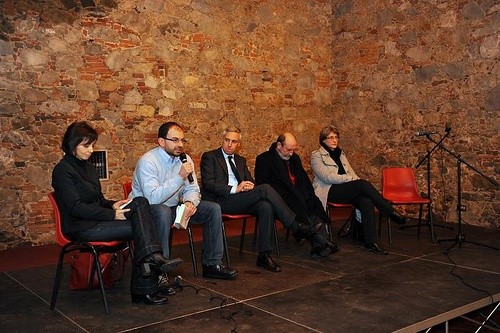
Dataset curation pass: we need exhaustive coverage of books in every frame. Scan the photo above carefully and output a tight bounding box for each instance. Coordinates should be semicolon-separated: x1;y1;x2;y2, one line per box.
175;204;189;229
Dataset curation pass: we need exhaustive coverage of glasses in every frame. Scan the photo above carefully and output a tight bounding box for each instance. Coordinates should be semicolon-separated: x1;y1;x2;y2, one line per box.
324;136;339;140
164;137;187;144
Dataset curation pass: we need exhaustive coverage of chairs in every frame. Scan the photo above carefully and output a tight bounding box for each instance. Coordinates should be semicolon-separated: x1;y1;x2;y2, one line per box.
48;167;432;317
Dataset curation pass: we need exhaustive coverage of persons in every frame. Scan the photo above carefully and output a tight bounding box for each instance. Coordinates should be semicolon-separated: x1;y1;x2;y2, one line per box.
52;122;184;305
254;133;340;257
200;125;339;272
310;125;407;255
128;121;239;293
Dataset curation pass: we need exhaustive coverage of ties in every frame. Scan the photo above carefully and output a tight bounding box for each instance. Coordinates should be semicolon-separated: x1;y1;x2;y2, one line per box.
228;156;242;185
286;160;296;187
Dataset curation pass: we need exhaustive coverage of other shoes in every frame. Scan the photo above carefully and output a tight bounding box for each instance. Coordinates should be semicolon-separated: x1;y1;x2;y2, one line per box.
310;240;339;259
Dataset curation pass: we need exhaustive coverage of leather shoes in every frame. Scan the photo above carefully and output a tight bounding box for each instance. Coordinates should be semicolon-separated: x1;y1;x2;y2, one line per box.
203;264;240;280
391;211;405;224
257;255;281;272
160;284;176;295
293;223;323;247
365;242;388;255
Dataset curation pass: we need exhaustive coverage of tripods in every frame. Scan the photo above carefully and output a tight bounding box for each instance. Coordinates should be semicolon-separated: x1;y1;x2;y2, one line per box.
399;127;500;254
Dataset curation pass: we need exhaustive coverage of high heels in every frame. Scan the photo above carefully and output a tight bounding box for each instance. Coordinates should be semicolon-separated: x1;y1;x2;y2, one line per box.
131;293;168;305
138;252;182;280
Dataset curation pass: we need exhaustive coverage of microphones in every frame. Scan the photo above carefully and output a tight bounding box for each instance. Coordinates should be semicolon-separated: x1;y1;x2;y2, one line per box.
414;131;438;136
175;275;183;288
180;153;194;182
